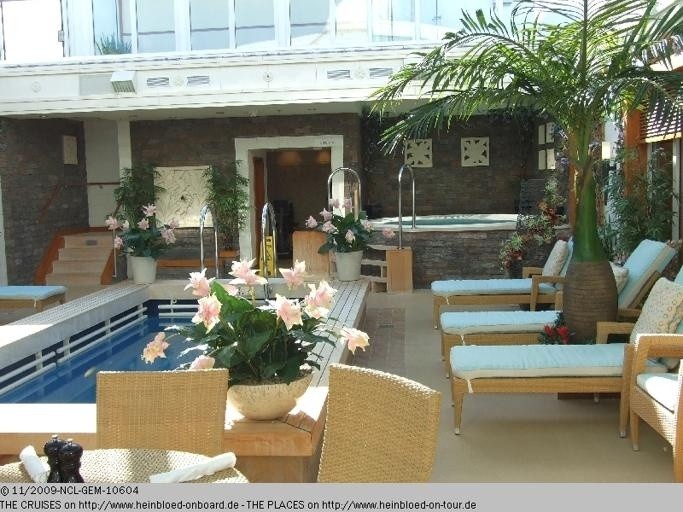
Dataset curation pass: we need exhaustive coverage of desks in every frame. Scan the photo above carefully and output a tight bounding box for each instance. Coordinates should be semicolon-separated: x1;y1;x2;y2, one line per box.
1;285;68;313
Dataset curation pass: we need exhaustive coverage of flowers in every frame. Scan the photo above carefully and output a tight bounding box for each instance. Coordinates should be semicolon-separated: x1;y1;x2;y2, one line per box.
306;198;396;253
105;201;180;256
143;254;372;385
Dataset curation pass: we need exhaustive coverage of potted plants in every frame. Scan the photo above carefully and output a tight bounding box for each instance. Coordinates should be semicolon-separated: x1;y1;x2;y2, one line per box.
112;157;166;280
499;176;569;311
201;158;256;266
365;0;683;342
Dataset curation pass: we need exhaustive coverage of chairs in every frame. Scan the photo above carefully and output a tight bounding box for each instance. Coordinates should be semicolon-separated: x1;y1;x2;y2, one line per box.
450;266;683;435
317;364;443;483
439;239;678;379
630;333;683;482
430;236;574;330
95;369;228;453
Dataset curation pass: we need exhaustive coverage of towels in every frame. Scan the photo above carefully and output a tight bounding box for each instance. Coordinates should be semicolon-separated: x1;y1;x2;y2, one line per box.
150;452;236;483
19;444;49;483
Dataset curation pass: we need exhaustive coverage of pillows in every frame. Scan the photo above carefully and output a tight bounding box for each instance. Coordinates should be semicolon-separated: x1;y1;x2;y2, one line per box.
629;277;683;361
542;239;567;286
609;260;628;294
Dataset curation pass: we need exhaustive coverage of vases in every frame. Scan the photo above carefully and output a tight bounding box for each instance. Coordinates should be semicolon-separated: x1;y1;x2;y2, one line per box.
333;251;362;282
131;255;157;285
231;365;317;419
125;253;137;280
